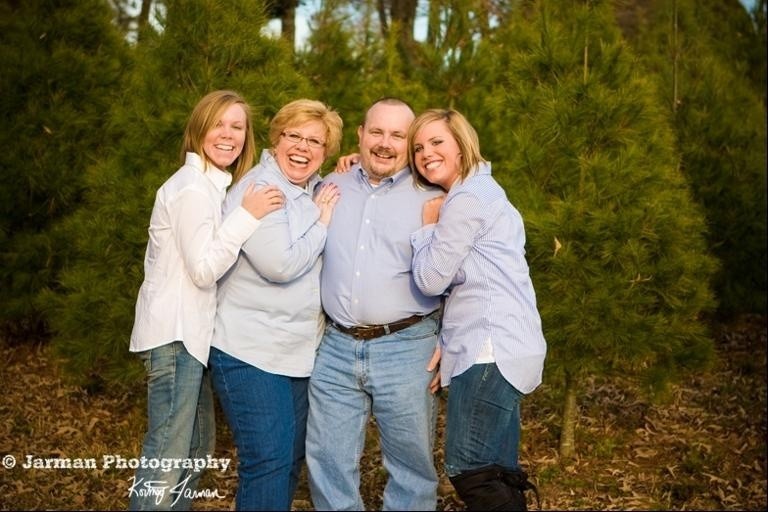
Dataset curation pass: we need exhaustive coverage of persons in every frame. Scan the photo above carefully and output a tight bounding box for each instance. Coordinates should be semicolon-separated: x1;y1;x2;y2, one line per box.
128;89;291;511
310;94;448;511
210;97;345;510
331;105;551;510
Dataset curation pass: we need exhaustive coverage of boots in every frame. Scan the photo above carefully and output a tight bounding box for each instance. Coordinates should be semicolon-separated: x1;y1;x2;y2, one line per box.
449;464;539;511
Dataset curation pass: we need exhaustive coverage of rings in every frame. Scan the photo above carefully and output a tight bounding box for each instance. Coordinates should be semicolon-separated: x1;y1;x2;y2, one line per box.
322;199;326;203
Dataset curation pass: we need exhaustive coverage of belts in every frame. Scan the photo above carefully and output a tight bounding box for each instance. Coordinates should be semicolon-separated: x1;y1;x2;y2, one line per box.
330;310;436;340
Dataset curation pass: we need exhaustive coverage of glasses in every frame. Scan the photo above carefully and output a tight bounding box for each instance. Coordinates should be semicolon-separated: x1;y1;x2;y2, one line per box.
280;130;327;149
